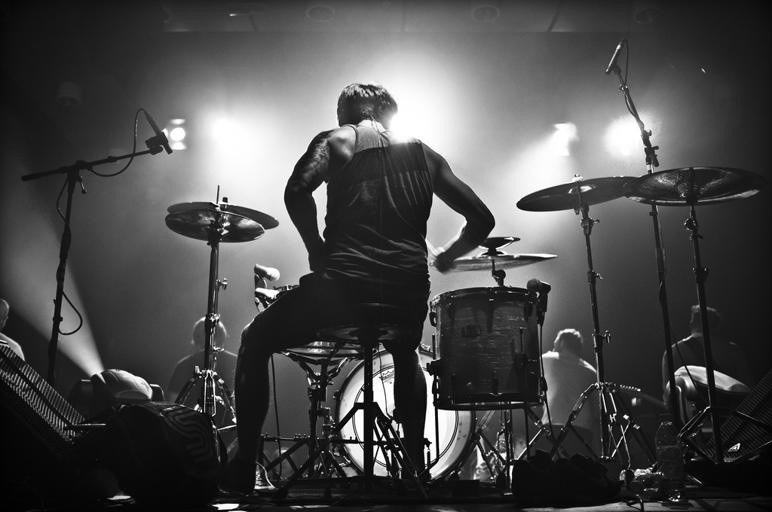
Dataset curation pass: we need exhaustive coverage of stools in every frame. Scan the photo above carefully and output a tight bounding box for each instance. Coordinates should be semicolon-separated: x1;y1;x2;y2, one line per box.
329;301;426;498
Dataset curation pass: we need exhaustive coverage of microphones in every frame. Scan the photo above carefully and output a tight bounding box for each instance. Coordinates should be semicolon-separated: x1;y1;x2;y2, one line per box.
605;41;624;74
143;108;173;154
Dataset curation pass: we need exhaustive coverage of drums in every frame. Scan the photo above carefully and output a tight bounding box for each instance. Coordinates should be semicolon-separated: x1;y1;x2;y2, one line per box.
271;284;363;360
426;286;547;410
333;342;480;479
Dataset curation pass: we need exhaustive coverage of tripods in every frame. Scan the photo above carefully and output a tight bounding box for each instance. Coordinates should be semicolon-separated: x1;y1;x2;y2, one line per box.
537;203;670;505
658;207;770;502
470;409;541;502
173;229;238;463
269;355;366;507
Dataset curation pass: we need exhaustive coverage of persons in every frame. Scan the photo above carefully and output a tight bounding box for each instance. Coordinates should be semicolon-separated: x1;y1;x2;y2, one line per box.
0;298;25;362
220;82;495;495
165;316;237;403
662;304;741;416
539;328;600;451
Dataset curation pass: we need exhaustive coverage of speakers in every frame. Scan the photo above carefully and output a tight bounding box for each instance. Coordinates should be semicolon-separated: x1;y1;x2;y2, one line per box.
0;345;122;512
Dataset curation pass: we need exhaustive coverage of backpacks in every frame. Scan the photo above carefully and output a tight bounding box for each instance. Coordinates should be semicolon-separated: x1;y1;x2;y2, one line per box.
105;398;229;512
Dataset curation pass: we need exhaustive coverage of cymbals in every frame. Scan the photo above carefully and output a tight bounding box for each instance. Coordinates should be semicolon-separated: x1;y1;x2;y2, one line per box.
476;236;521;248
165;202;278;243
517;177;636;212
622;167;770;207
448;251;556;272
